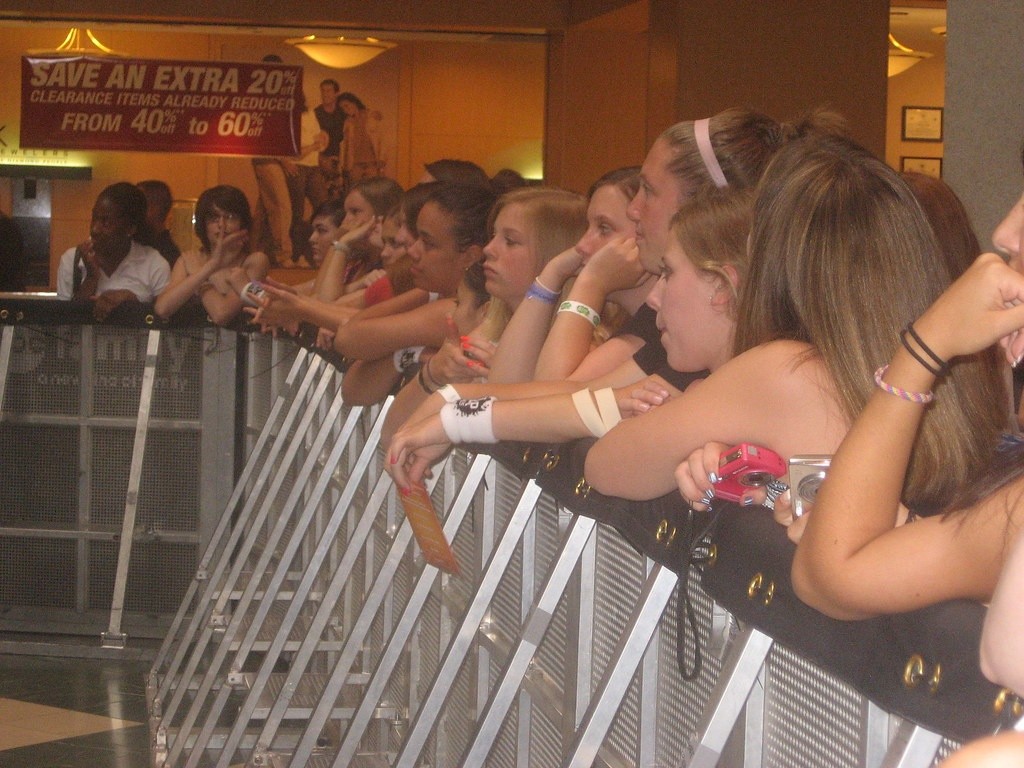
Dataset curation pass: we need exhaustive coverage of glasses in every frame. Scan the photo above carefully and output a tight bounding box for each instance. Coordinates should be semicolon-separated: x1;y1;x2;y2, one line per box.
204;211;243;225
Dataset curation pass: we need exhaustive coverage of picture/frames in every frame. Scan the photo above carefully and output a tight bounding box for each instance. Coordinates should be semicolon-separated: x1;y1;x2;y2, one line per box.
901;105;944;143
899;155;942;178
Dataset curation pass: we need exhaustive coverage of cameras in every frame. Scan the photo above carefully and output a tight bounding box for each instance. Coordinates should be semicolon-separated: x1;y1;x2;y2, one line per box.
714;444;787;502
789;455;834;517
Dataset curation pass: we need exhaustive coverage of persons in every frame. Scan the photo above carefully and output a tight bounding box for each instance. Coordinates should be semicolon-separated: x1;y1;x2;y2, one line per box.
0;106;1024;768
249;53;387;267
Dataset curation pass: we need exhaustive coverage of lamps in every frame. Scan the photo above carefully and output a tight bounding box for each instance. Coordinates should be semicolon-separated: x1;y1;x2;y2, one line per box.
25;27;130;57
888;33;935;77
285;35;396;70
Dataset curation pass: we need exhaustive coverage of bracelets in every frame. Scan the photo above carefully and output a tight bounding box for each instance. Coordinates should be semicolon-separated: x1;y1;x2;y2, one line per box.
558;299;601;329
440;395;500;446
418;368;433;394
426;356;444;386
899;329;944;377
331;240;338;245
437;383;461;403
535;275;562;295
872;366;934;403
907;322;949;368
393;344;426;373
526;281;560;304
335;244;351;253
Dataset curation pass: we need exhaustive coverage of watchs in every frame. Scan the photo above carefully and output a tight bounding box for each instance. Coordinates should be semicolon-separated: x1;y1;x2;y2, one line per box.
762;478;788;511
